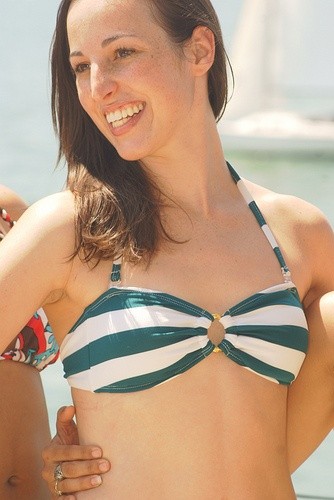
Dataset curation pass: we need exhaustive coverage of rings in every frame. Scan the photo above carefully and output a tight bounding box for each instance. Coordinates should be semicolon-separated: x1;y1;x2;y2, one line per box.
54;462;66;480
54;479;62;497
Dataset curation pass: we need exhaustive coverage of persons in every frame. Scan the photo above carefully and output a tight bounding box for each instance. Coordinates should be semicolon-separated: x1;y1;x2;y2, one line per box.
2;188;110;498
2;0;334;498
